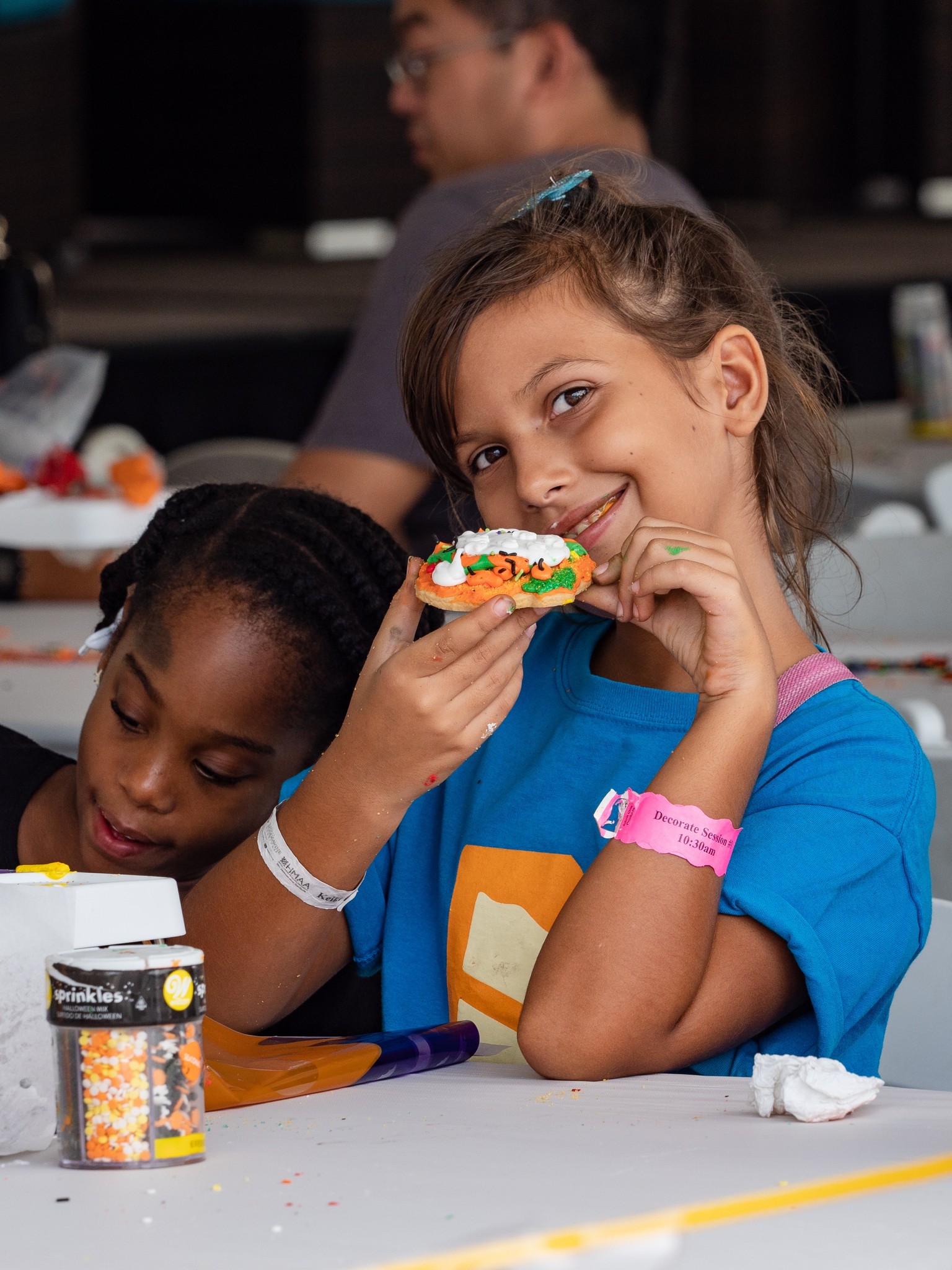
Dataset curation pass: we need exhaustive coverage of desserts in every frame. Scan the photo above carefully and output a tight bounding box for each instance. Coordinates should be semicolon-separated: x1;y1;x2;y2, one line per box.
414;525;595;610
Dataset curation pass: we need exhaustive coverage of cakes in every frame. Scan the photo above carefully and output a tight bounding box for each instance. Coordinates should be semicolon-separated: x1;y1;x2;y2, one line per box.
1;426;200;600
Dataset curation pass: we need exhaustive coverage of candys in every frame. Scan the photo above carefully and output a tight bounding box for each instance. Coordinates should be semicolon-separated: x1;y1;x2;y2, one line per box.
60;1025;203;1165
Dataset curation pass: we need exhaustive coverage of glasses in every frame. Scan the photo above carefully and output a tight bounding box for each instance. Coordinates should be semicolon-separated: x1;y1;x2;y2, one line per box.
387;31;502;83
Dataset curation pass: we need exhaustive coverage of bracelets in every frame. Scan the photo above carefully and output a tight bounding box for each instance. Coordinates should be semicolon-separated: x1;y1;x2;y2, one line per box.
256;796;368;913
590;788;741;878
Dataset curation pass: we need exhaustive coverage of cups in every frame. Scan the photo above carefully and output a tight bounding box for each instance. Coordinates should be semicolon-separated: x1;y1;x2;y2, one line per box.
889;280;951;426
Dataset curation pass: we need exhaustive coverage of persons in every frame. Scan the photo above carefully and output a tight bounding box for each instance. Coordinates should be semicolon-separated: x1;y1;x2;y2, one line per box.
0;485;443;1041
279;3;738;562
167;167;934;1087
